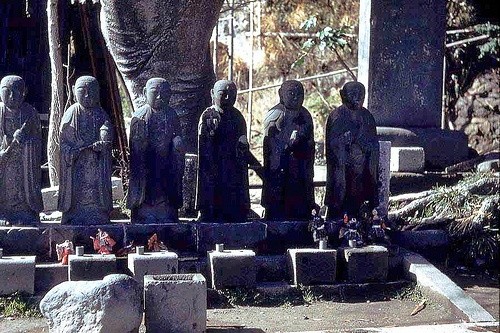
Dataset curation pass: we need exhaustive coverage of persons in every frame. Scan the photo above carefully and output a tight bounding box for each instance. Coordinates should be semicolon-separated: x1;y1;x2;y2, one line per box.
126;77;184;224
261;81;315;220
326;81;379;220
0;76;43;226
197;80;250;222
57;76;113;226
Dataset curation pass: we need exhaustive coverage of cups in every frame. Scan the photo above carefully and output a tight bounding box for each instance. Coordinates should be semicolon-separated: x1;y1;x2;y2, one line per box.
76;245;84;256
215;243;224;252
136;246;145;255
0;248;3;258
348;239;356;248
319;240;327;250
